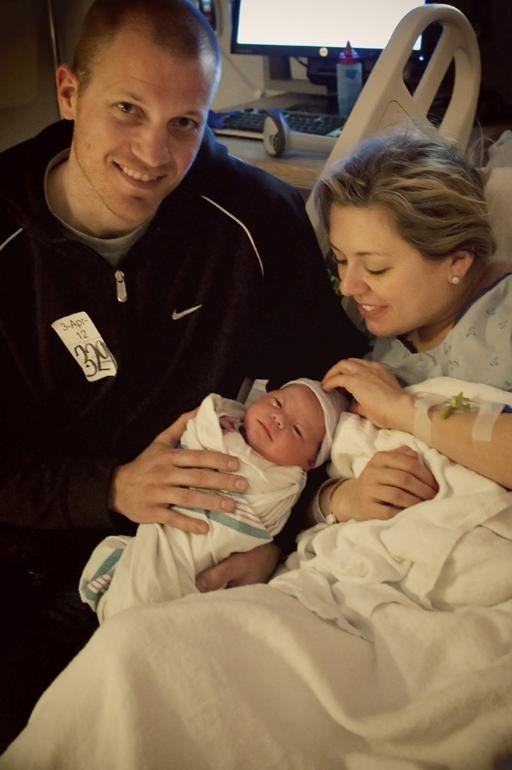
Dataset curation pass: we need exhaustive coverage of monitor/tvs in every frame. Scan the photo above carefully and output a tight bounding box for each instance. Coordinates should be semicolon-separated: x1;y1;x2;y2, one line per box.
229;0;429;61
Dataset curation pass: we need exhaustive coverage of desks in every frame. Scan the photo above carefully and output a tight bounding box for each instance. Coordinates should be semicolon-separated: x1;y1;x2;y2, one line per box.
212;92;507;200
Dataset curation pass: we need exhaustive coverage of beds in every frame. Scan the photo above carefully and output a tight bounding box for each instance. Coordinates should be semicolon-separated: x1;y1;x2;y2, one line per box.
234;7;512;260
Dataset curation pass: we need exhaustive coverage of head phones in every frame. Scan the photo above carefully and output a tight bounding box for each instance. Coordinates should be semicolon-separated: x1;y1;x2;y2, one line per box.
262;111;338;156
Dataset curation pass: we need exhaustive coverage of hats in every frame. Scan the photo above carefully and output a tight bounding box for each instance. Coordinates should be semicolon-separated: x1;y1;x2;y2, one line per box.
283;372;349;468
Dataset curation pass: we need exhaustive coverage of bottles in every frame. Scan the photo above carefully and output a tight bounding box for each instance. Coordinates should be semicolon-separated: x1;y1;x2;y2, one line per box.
338;42;362;118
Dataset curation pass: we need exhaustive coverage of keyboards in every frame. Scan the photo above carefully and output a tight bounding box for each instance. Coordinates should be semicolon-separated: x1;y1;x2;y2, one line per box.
208;105;349;147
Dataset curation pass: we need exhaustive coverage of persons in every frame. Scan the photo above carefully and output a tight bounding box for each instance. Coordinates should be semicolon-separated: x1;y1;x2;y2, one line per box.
99;379;348;618
0;136;512;770
0;0;371;758
